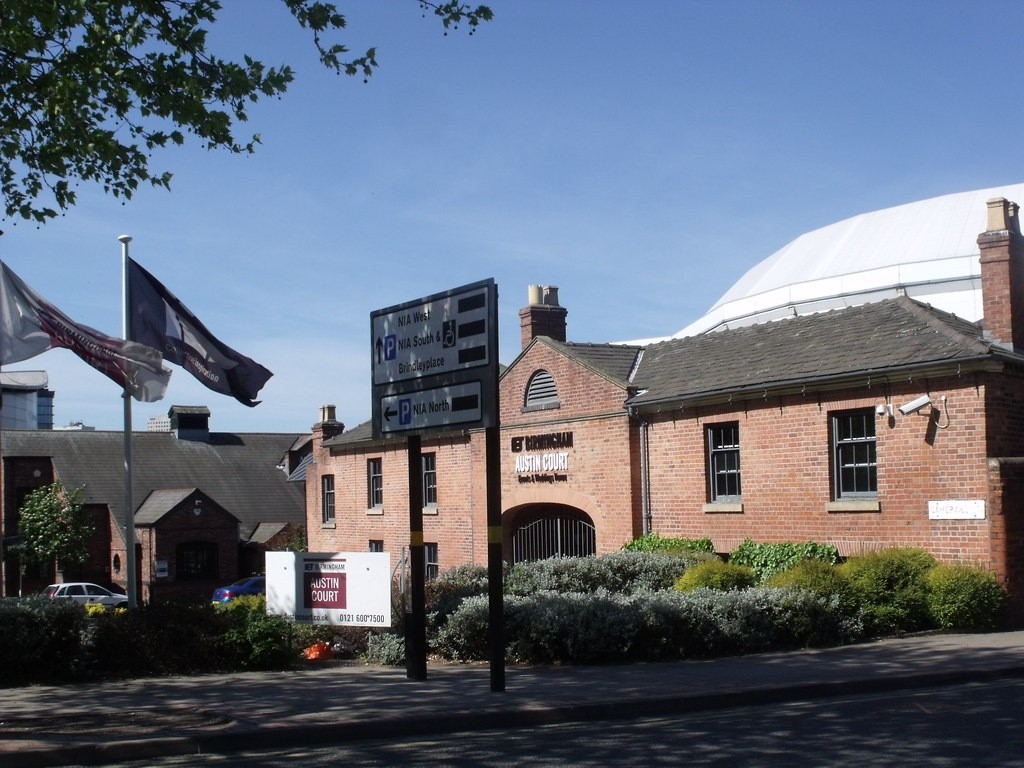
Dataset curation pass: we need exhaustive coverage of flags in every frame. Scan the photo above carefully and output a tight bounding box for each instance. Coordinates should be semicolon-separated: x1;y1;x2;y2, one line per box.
127;260;272;407
0;260;171;403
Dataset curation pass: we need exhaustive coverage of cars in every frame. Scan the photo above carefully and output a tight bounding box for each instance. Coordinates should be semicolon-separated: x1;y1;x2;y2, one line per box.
44;583;129;608
210;577;265;605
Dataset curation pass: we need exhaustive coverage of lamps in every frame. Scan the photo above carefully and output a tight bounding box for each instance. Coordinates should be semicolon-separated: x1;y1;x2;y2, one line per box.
194;500;203;515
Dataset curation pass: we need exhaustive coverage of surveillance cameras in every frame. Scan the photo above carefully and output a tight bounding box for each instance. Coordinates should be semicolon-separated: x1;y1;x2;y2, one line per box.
898;395;932;416
